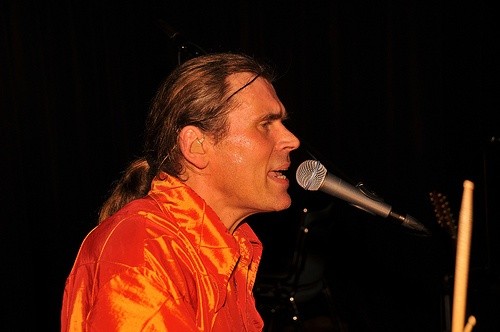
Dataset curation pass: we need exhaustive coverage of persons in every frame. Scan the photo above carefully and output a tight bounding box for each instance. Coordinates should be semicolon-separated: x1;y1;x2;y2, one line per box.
58;52;301;332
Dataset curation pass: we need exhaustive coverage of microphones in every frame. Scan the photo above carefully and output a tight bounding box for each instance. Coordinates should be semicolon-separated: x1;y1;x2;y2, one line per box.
296;160;425;232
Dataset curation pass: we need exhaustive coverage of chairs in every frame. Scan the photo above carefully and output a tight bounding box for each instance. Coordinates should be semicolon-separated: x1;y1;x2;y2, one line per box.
258;212;319;288
258;225;341;332
